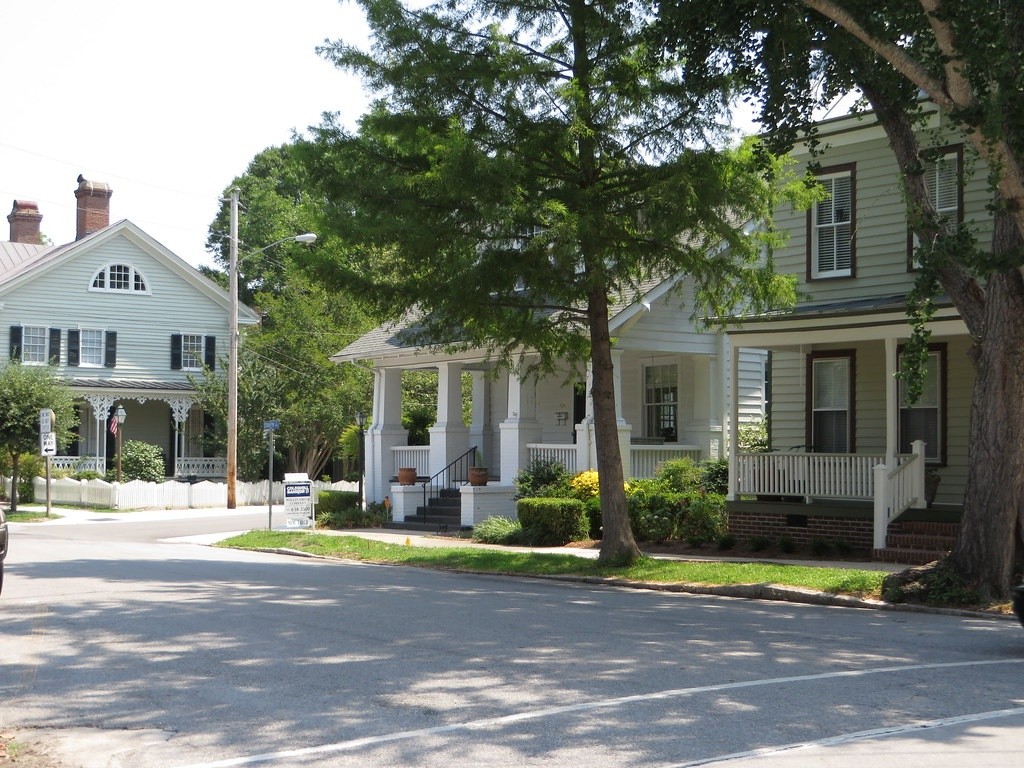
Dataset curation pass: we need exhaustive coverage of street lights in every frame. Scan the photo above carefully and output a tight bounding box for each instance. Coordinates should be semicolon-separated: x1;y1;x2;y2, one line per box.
227;233;318;509
113;403;127;484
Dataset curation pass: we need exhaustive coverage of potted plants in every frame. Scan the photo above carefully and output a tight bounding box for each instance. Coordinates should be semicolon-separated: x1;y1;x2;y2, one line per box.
739;414;774;500
468;451;490;486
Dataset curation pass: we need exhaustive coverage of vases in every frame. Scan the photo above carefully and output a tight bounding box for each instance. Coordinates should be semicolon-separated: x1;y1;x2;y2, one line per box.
398;467;416;486
